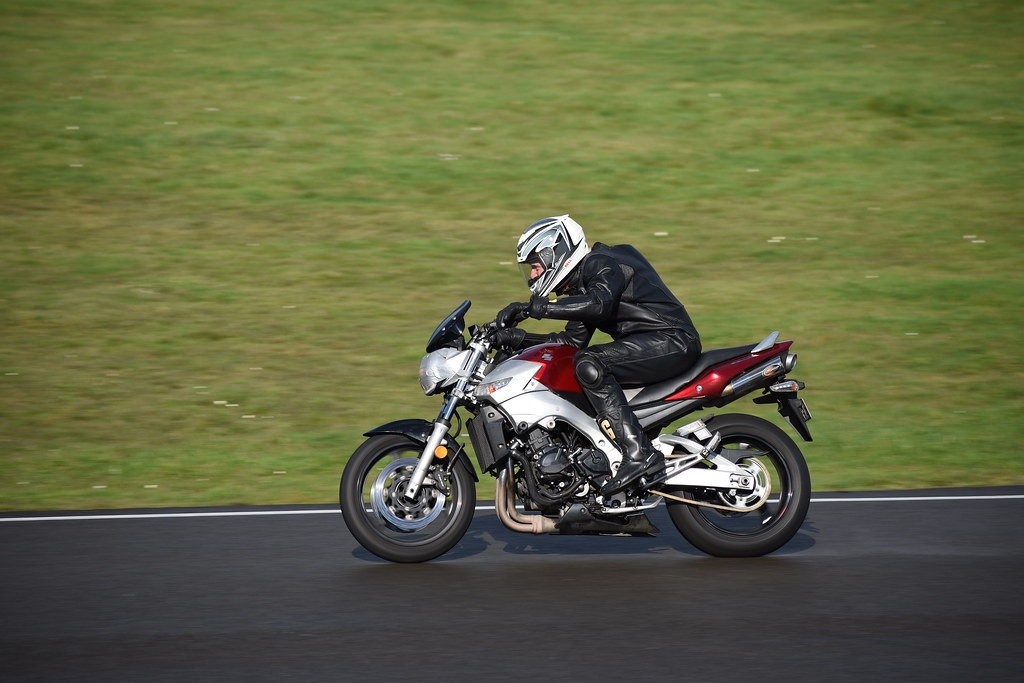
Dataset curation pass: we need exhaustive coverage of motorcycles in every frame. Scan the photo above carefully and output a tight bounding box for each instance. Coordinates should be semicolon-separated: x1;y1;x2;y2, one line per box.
339;299;814;564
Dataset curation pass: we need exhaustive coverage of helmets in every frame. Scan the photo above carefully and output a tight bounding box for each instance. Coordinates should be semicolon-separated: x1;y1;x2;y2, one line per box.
516;213;591;298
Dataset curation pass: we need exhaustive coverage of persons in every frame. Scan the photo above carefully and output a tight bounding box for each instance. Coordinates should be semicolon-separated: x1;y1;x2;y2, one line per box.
487;214;702;496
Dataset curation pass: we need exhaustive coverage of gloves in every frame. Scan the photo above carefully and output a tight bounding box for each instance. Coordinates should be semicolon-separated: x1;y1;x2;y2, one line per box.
496;301;533;331
486;327;526;354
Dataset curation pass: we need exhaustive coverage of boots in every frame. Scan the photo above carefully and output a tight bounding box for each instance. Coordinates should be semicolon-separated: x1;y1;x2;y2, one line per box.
596;405;666;495
